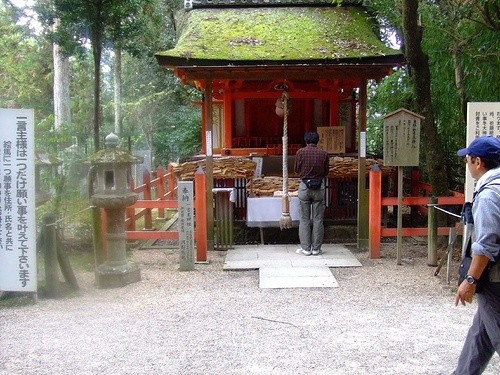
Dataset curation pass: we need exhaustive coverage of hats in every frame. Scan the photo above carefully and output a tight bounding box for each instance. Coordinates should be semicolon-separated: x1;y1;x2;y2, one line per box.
457;136;500;156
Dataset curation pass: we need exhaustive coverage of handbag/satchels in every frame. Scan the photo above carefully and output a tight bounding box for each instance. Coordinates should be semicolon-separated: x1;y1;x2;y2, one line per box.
458;256;489;293
302;177;323;189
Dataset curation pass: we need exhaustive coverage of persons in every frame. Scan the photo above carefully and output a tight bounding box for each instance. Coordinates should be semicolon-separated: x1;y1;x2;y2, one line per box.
296;132;329;256
451;136;500;375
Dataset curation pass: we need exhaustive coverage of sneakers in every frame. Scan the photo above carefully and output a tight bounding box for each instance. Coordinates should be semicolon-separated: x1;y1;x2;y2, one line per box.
311;248;319;254
297;248;311;255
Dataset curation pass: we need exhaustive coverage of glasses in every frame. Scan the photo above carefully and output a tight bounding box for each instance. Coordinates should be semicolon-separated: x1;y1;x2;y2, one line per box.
464;157;474;163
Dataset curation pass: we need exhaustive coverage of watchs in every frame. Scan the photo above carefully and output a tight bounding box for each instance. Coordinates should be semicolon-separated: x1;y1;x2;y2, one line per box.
463;274;479;285
